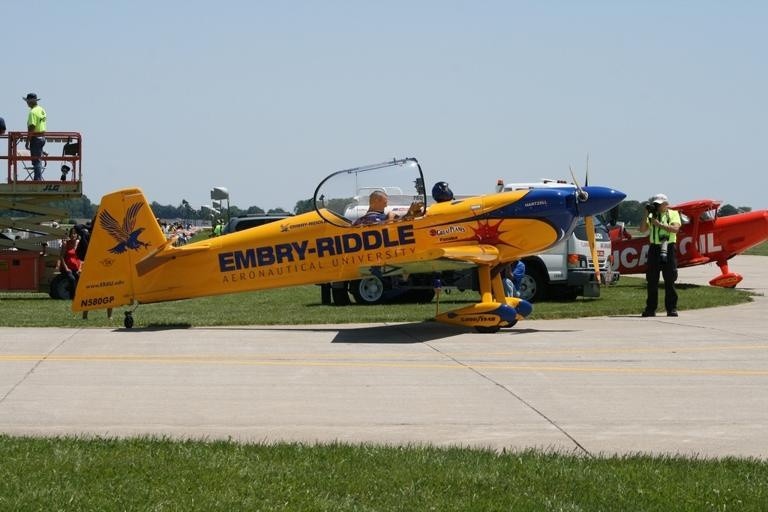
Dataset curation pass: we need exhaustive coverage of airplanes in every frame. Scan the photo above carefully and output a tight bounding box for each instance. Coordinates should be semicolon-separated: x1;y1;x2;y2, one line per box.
612;197;767;290
70;157;628;338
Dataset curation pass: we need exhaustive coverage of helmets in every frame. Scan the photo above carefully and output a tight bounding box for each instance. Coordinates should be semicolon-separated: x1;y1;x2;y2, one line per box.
431;181;453;203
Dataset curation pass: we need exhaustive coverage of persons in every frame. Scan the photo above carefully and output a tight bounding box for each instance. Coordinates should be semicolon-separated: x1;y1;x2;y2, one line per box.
213;219;223;236
22;93;46;181
639;193;682;317
221;218;225;231
502;259;525;297
156;218;197;247
364;191;400;225
74;216;113;320
60;227;82;299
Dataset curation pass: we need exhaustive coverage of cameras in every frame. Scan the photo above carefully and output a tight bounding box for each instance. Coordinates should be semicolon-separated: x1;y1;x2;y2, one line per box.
646;205;658;219
659;234;669;264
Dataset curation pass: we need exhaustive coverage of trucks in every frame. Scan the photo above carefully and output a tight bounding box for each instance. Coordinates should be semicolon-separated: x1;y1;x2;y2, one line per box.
342;178;611;303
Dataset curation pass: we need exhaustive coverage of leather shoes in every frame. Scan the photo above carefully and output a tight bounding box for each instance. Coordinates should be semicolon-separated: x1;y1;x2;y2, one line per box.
666;311;678;316
642;310;655;317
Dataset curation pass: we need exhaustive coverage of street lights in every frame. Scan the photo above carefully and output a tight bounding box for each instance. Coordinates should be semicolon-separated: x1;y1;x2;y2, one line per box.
199;184;231;242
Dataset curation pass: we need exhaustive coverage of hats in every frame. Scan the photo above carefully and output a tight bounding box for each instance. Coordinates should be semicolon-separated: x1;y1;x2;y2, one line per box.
652;194;668;206
22;93;40;101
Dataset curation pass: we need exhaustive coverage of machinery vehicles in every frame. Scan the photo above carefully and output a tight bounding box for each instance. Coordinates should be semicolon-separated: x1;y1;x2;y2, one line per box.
1;129;85;301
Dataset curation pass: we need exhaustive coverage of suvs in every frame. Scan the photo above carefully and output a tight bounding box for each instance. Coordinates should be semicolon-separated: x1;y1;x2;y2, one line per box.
222;211;293;235
